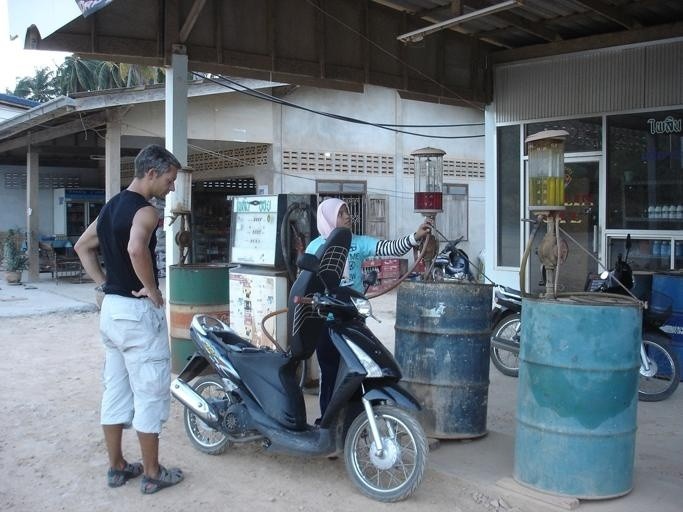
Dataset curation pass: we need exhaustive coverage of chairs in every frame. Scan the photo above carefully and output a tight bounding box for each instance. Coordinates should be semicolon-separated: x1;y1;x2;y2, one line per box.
49;249;83;283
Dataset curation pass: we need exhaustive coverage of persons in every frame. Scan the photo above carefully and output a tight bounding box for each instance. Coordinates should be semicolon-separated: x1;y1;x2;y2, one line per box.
299;196;435;463
70;143;185;496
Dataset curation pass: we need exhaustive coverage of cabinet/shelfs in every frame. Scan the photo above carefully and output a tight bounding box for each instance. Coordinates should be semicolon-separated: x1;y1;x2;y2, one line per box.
621;173;683;269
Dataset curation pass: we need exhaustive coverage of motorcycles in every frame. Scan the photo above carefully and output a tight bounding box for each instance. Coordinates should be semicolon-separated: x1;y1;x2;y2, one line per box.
487;232;681;402
169;253;430;502
409;235;478;284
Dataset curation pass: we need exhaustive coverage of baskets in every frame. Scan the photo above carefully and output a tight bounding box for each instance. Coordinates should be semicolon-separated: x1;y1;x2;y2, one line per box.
638;290;673;327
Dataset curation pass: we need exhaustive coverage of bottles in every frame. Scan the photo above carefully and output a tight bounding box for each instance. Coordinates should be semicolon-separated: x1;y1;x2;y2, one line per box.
653;240;683;255
648;203;683;218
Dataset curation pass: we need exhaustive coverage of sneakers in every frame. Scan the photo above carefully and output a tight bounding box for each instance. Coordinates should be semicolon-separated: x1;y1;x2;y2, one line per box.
108;462;143;487
141;464;183;494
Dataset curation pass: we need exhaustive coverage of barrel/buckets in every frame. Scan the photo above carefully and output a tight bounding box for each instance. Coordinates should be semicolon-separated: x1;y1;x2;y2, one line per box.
515;292;641;500
632;268;651;309
395;281;492;439
650;271;679;380
170;263;231;375
380;257;400;285
